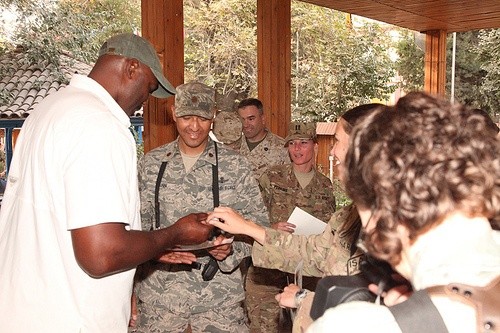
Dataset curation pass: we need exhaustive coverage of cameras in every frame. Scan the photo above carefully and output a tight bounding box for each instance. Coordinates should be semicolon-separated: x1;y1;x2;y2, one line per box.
309;254;397;322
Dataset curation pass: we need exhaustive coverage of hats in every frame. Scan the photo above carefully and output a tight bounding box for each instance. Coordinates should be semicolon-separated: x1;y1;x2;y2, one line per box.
212;112;243;150
174;81;215;120
98;33;178;99
284;118;316;147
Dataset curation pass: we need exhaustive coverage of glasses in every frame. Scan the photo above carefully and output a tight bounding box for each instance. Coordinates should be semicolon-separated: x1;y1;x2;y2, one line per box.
355;227;371;255
